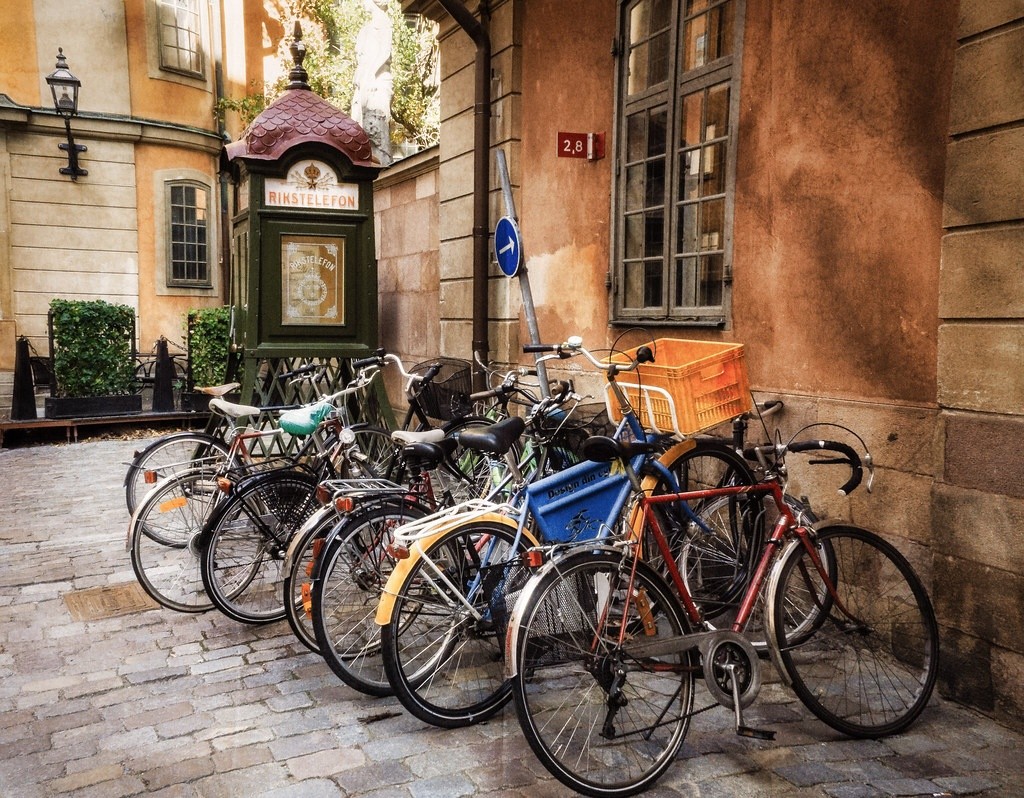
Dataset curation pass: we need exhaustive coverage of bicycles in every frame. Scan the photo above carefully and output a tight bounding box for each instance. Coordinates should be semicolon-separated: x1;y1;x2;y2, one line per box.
122;325;939;798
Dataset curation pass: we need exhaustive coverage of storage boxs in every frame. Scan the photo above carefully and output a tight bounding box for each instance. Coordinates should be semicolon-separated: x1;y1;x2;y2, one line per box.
595;336;750;439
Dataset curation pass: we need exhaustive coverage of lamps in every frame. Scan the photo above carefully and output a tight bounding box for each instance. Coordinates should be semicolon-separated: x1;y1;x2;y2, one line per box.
46;45;93;183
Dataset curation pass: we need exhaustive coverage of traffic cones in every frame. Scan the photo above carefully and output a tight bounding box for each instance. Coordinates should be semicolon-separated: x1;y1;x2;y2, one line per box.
11;334;38;421
150;336;177;411
